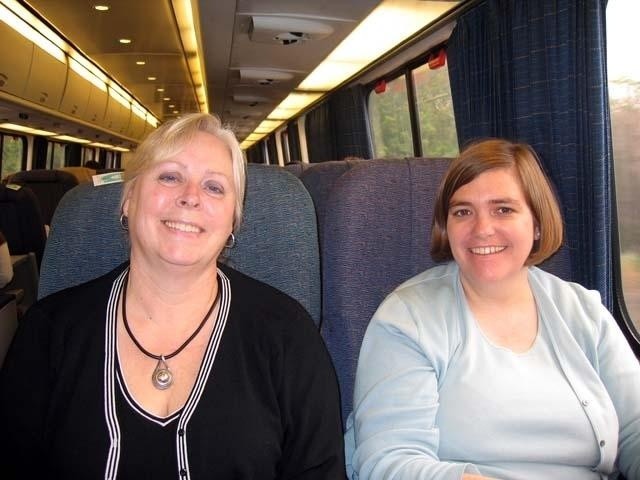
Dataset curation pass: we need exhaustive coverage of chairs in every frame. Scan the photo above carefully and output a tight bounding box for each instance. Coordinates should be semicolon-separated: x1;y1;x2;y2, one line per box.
1;157;465;434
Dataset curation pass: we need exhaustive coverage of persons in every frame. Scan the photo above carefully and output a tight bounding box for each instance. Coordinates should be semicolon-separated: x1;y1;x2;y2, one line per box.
0;108;347;480
344;134;640;480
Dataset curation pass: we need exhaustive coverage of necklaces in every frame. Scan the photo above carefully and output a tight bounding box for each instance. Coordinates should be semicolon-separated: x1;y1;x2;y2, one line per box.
120;270;222;390
